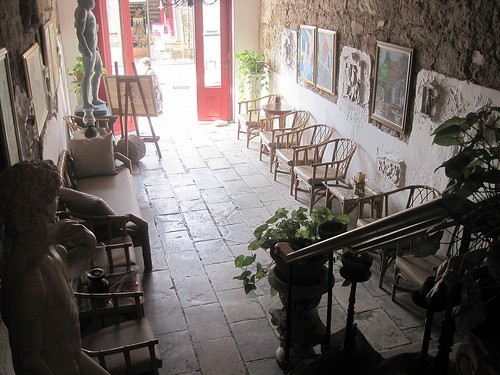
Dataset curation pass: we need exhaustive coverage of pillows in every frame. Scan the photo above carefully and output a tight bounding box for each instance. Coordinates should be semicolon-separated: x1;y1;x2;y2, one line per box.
59;186;123;230
67;133;119;179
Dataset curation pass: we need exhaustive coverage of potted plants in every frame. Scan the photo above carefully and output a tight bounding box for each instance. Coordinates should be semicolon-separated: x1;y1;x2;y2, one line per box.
235;48;272;102
233;206;351;326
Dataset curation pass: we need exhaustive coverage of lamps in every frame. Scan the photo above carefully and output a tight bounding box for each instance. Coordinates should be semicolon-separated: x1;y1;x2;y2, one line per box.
83;108;97;139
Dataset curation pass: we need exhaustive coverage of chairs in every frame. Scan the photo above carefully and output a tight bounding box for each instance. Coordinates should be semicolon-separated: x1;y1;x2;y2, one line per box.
51;210;163;375
63;115;113;134
236;94;357;216
356;184;495;323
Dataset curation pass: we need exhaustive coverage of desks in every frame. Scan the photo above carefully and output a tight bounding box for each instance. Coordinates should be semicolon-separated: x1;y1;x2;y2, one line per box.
321;177;384;220
73;128;113;141
73;271;145;337
263;102;294;131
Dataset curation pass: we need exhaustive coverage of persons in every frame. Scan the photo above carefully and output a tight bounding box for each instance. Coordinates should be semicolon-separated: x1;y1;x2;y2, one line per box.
140;57;164;115
0;159;112;375
74;0;108;109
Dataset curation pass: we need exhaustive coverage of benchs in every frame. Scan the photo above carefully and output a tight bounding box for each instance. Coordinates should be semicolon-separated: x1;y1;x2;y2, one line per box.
53;150;153;273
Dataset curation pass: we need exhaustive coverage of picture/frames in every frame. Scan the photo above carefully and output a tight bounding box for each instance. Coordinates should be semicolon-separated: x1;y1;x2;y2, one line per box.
315;28;337;95
298;24;317;86
22;42;49;141
0;47;24;166
41;19;60;99
370;41;414;134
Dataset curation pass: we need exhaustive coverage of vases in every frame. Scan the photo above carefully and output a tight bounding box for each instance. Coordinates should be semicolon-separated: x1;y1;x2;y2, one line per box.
87;267;111;308
